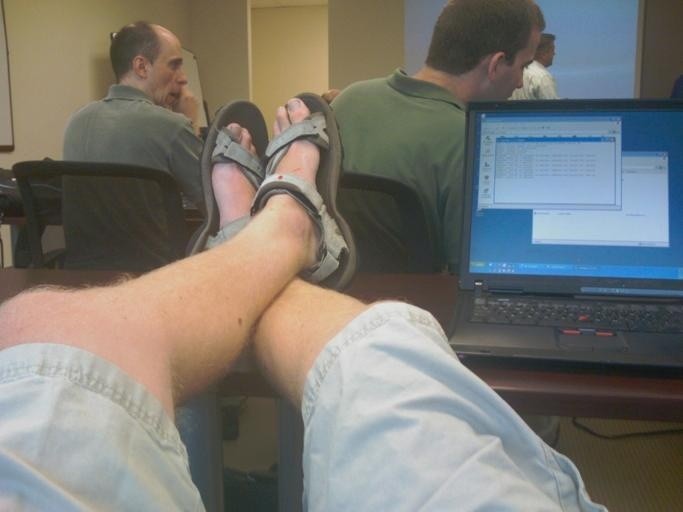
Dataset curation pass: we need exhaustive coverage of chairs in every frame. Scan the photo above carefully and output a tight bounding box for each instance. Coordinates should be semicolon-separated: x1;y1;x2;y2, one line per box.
336;169;437;274
12;157;187;273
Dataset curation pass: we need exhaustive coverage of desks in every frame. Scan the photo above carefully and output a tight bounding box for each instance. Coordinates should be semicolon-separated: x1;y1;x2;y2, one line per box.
1;268;683;512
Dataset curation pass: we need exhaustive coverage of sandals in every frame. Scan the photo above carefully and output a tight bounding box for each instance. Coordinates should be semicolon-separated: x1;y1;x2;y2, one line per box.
184;91;358;291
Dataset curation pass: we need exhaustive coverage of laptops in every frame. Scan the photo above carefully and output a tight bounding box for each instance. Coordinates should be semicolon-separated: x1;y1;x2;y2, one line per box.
443;96;683;377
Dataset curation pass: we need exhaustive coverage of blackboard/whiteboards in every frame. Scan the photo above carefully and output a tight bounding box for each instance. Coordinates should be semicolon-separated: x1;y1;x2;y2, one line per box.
0;0;15;152
111;32;210;136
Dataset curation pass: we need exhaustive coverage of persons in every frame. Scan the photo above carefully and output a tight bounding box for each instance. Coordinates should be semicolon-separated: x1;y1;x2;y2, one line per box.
56;18;206;270
0;90;614;512
505;30;562;100
314;2;546;280
